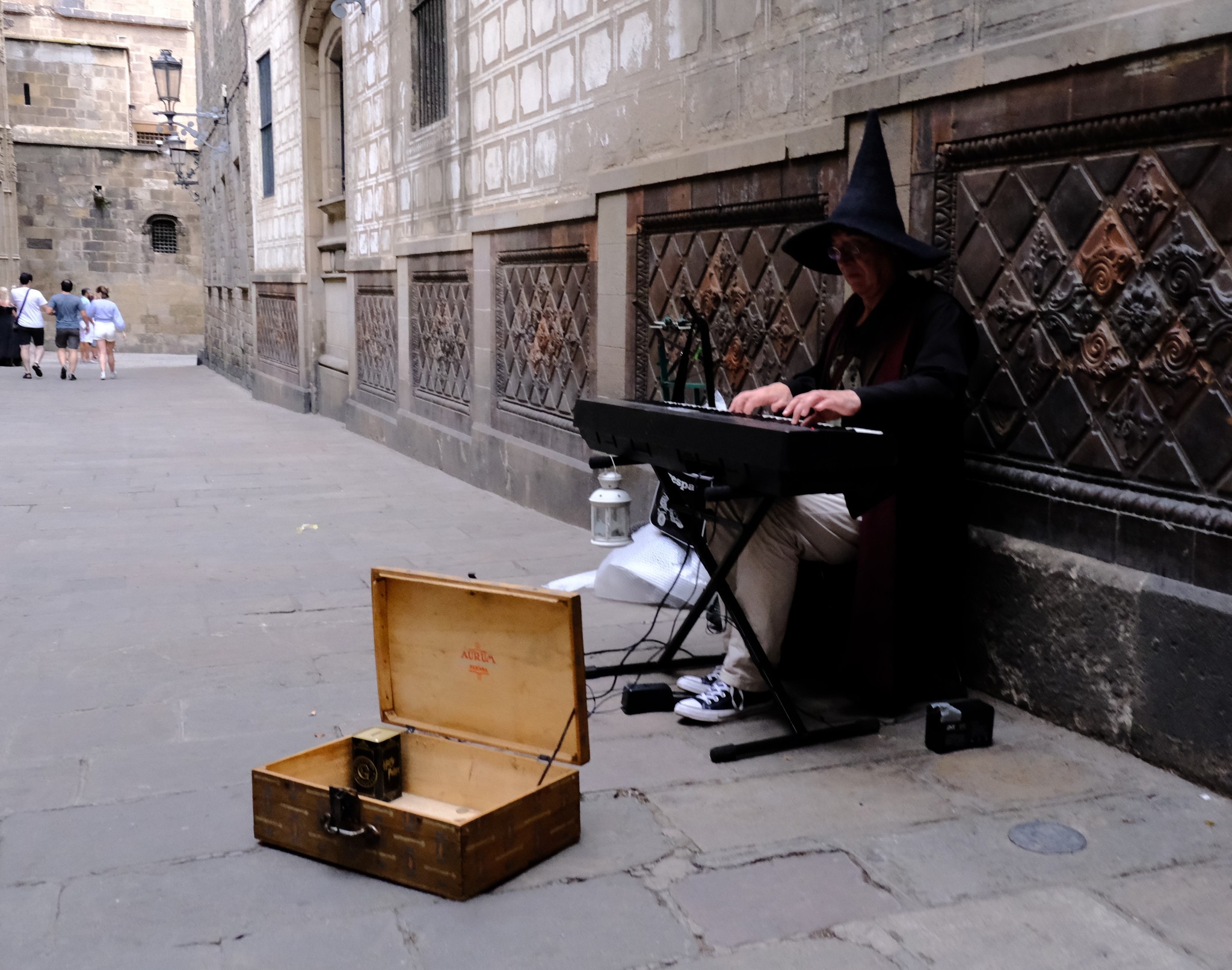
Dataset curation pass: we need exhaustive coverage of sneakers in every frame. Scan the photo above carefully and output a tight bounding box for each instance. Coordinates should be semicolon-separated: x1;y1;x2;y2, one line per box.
674;678;764;722
677;664;724;693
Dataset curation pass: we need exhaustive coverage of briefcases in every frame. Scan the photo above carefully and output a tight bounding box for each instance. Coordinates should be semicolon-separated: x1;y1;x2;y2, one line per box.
253;568;591;903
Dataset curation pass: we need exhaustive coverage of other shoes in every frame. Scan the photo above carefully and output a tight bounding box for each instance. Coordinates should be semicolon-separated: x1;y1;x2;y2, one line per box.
100;372;106;380
111;371;118;378
60;365;67;379
69;373;76;380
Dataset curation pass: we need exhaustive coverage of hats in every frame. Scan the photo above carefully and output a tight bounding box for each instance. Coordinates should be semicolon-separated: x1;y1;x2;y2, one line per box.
782;109;948;276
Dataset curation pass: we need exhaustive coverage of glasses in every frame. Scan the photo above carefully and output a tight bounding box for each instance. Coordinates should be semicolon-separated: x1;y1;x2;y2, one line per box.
828;242;861;259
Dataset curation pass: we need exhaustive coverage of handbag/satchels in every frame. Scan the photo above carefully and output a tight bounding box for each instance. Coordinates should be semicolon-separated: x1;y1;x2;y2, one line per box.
12;318;18;329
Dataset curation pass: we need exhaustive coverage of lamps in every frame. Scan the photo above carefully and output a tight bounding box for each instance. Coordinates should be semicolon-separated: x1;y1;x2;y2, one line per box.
148;49;229;153
164;130;200;201
588;454;634;547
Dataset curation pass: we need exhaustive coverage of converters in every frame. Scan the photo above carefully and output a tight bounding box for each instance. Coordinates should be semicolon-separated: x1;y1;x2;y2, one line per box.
621;683;674;714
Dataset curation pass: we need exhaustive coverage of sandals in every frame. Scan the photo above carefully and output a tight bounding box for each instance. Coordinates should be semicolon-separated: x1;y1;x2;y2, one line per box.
23;372;32;379
32;362;43;377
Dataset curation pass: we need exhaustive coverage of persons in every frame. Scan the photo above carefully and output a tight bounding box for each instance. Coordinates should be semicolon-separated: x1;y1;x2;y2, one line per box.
672;106;976;723
78;287;99;364
46;278;90;381
0;285;22;367
11;273;55;379
87;285;127;380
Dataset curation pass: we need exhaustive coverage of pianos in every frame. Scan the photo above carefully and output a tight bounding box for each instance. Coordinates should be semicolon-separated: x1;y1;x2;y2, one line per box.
573;396;883;765
